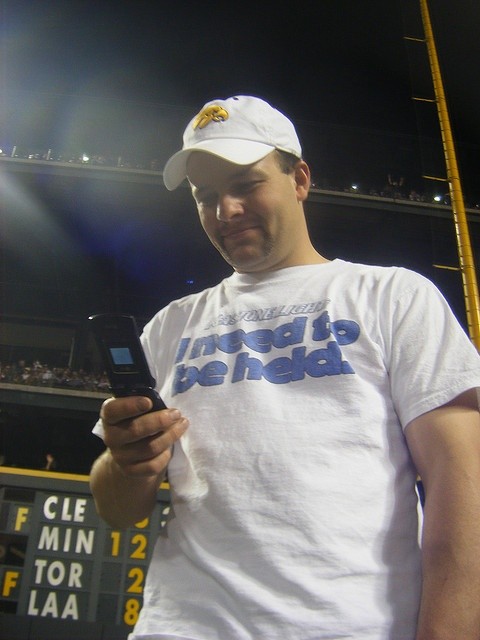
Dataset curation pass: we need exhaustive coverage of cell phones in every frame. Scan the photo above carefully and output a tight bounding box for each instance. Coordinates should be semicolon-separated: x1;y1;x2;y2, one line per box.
87;312;168;414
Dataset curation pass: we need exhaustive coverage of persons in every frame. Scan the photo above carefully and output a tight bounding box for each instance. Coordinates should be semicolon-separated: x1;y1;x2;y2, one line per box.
90;96;480;639
43;449;71;473
310;181;451;206
0;142;169;174
0;357;113;395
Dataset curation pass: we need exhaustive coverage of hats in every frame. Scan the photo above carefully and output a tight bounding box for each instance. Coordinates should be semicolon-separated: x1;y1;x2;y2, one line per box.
163;95;302;192
